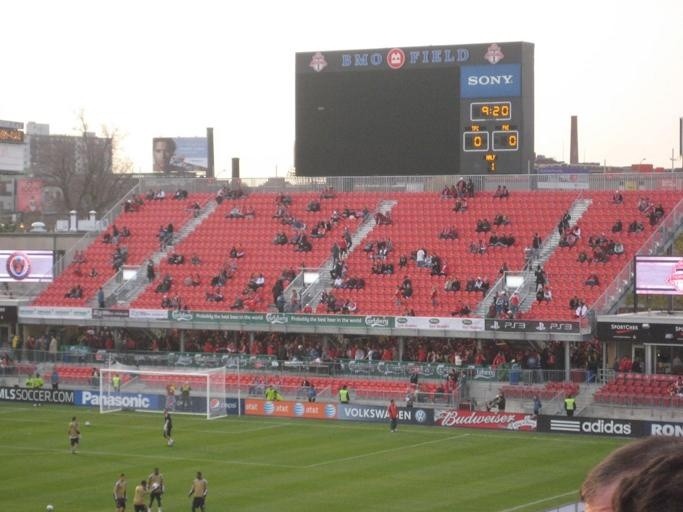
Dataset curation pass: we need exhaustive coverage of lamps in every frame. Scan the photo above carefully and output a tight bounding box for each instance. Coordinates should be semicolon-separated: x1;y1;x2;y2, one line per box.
593;372;681;408
502;381;579;401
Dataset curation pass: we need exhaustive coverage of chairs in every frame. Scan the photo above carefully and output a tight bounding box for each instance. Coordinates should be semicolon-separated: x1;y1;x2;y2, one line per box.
29;188;681;325
593;372;681;408
502;381;579;401
10;359;466;405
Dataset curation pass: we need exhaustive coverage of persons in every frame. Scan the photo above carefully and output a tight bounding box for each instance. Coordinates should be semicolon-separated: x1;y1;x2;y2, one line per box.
133;480;148;512
164;409;175;447
113;472;128;512
1;186;683;432
146;466;165;512
188;470;208;512
154;138;182;171
66;415;80;454
579;436;683;512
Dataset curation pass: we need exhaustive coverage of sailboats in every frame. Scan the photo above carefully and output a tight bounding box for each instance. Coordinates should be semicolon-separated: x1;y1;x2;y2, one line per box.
45;504;54;510
85;421;90;426
152;483;160;488
